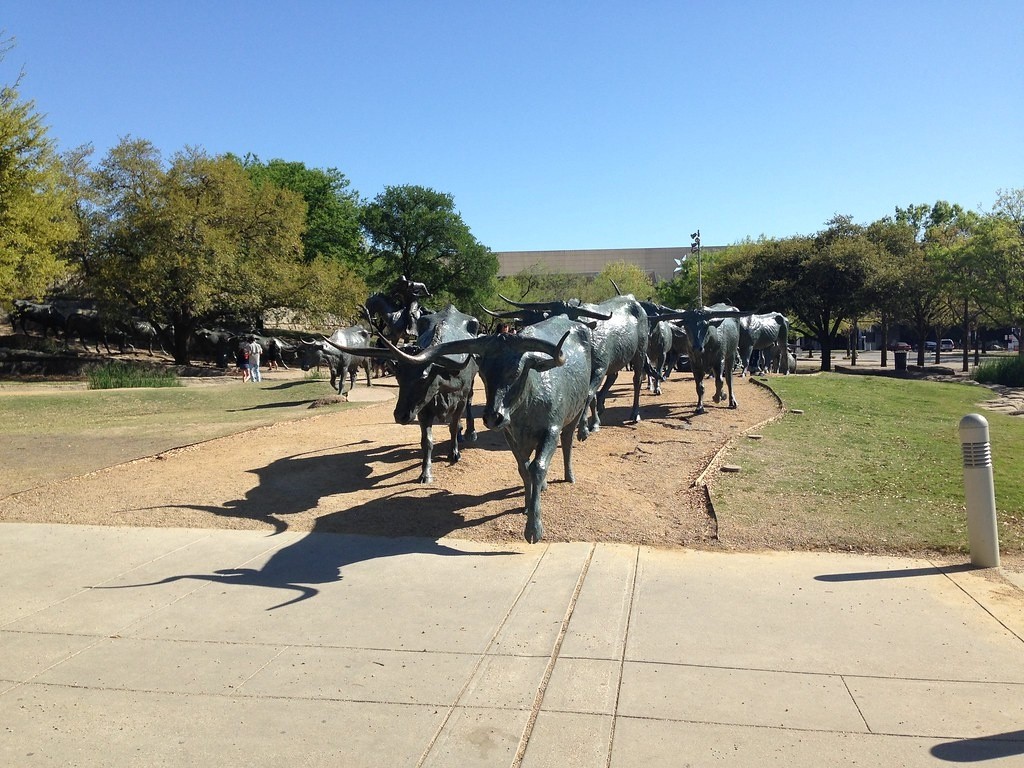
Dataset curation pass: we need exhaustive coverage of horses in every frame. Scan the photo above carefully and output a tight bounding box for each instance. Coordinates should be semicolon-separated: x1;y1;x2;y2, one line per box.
358;292;438;348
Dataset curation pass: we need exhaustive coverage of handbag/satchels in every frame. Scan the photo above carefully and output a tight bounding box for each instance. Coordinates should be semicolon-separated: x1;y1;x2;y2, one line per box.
250;353;259;365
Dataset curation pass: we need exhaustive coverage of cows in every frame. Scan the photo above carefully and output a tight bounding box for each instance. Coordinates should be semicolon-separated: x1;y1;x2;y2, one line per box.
280;303;374;395
319;278;798;546
8;299;308;371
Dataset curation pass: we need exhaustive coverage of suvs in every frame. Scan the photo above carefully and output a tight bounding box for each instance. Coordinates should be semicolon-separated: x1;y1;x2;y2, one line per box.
936;339;954;352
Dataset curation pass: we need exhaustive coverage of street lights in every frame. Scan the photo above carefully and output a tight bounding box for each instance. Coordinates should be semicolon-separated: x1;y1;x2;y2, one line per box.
690;229;702;309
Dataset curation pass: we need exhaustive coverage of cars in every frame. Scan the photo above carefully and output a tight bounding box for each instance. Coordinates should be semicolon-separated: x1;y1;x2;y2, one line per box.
890;341;911;351
991;344;1001;351
925;341;937;352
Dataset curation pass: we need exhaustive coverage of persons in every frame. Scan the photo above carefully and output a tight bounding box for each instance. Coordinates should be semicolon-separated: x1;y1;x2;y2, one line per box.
496;324;517;334
265;338;288;371
237;336;262;383
